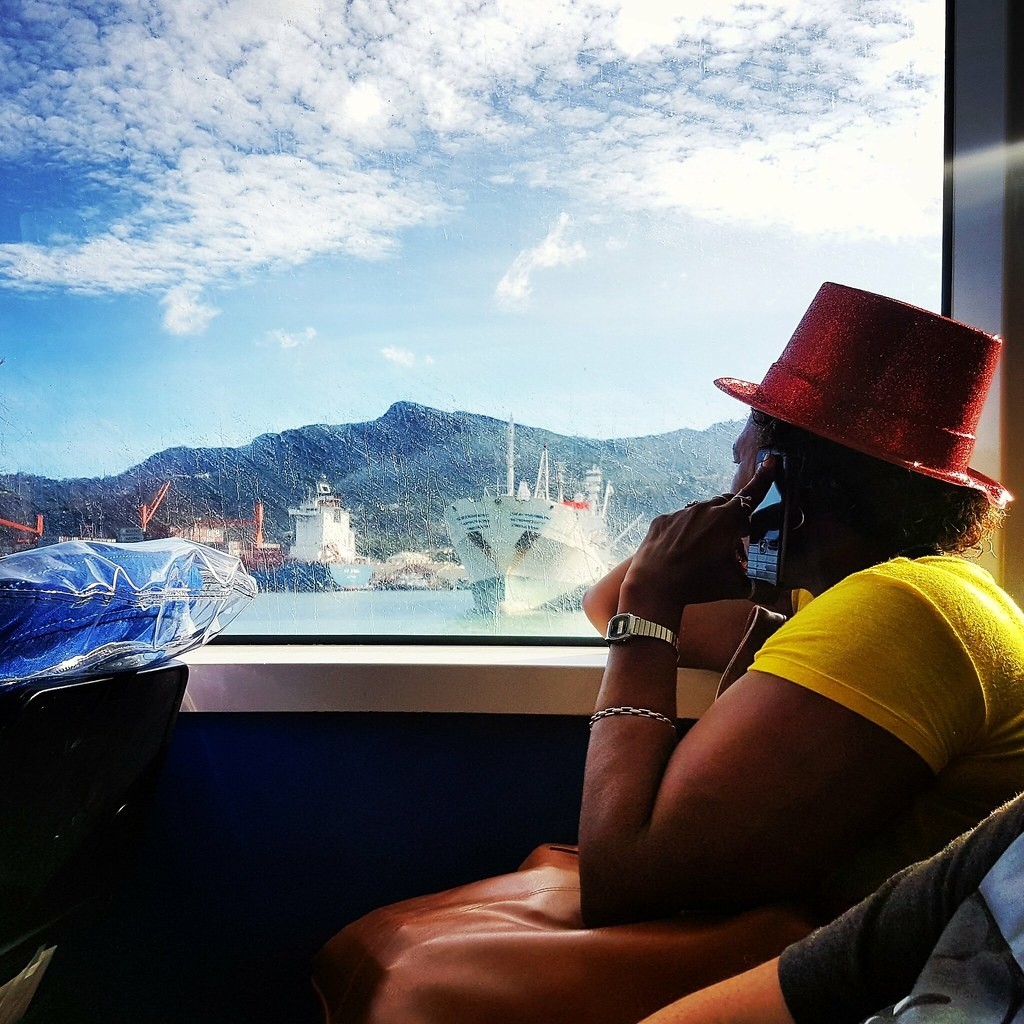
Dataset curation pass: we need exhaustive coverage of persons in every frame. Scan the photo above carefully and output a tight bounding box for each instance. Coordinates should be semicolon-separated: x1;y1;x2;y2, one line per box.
637;794;1024;1024
310;284;1023;1024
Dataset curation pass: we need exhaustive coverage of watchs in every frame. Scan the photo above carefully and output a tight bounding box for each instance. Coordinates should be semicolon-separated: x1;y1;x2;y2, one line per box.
604;613;680;661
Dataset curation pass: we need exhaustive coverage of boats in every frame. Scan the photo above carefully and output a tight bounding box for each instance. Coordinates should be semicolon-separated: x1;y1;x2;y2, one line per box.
246;483;375;592
442;416;611;612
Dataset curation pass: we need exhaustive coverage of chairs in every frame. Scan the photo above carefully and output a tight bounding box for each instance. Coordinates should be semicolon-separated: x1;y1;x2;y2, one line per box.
0;659;189;1024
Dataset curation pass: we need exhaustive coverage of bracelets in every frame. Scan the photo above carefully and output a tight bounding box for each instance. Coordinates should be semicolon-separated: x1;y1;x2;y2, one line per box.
588;706;677;735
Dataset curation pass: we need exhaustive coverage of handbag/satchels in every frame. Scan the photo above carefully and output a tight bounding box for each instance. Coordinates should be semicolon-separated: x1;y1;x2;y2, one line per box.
310;604;821;1024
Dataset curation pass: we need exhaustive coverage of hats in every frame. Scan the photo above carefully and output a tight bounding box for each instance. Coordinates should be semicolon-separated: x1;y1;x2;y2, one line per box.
714;281;1016;504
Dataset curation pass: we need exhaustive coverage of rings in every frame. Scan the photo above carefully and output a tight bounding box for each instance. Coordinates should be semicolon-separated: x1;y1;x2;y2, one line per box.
684;500;700;508
712;494;729;502
734;495;753;512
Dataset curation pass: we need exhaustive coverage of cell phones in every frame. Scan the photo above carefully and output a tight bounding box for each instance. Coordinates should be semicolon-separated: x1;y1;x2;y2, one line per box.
746;451;790;585
